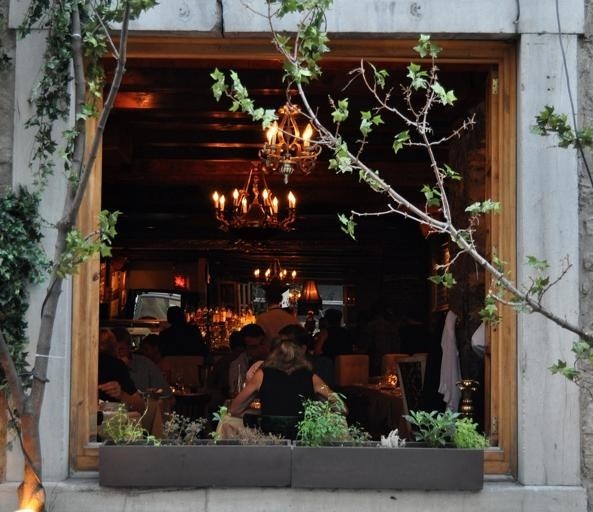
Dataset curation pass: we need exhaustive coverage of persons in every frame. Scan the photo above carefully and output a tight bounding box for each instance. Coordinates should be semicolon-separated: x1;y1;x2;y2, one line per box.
97;282;482;445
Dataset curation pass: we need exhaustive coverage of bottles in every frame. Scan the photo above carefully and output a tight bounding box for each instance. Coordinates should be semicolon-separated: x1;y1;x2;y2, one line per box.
234;364;246;396
186;303;257;348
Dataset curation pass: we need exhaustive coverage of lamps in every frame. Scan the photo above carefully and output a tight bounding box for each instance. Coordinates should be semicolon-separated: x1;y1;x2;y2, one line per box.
252;258;297;284
210;162;299;233
296;280;322;311
258;93;325;184
